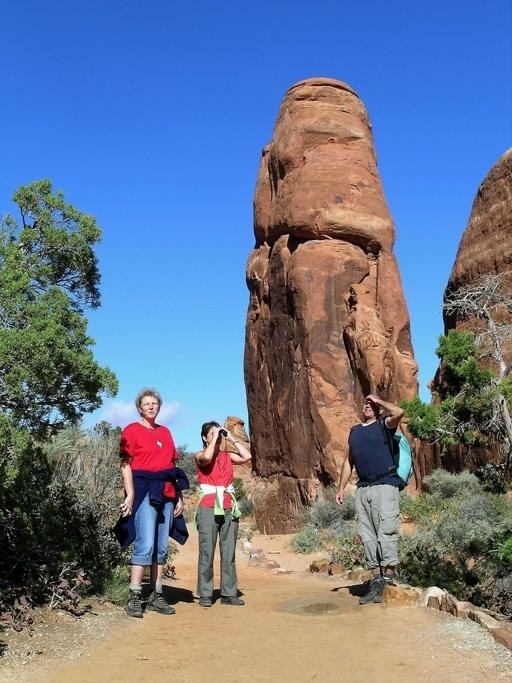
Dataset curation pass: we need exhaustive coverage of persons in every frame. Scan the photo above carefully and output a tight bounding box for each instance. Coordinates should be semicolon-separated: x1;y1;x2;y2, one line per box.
335;394;405;604
195;420;252;607
119;387;184;618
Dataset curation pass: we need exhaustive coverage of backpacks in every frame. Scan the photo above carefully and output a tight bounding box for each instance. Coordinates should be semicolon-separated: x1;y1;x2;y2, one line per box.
379;414;415;492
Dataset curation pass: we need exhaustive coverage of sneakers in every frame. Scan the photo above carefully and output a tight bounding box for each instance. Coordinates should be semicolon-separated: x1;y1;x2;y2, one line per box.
124;588;146;617
221;595;244;605
145;590;175;615
199;596;212;607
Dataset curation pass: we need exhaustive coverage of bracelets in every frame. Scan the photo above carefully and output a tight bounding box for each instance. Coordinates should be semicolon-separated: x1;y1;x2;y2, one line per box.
233;441;238;445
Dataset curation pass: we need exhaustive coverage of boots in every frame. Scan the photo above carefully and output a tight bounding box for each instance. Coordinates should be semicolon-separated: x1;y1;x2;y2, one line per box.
358;575;393;605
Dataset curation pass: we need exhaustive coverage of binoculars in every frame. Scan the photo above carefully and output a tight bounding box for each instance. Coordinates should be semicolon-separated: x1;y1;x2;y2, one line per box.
218;428;228;436
